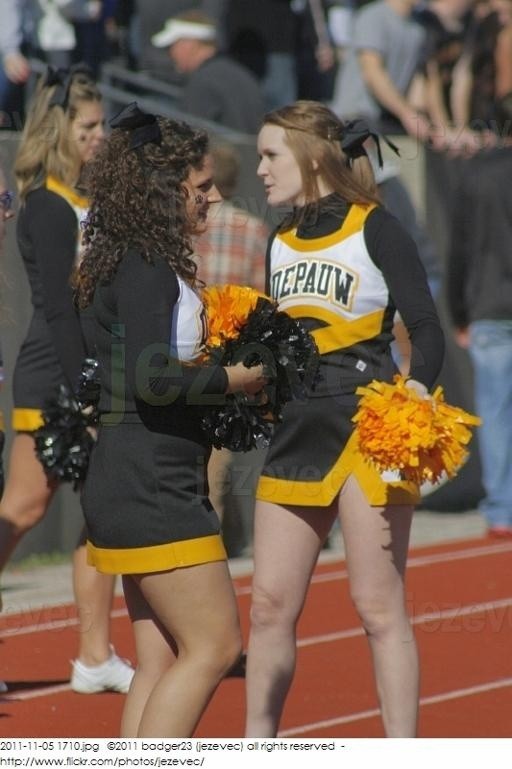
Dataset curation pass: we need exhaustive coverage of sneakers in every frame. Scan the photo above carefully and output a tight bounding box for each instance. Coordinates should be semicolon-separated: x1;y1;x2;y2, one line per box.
71;643;136;694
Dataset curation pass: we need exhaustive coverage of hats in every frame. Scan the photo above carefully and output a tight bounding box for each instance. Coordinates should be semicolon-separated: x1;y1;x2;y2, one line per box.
150;17;215;47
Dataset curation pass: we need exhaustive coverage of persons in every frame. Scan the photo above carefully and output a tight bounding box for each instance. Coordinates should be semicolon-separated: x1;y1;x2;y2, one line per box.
1;68;136;694
74;102;270;738
0;0;512;540
245;100;445;738
